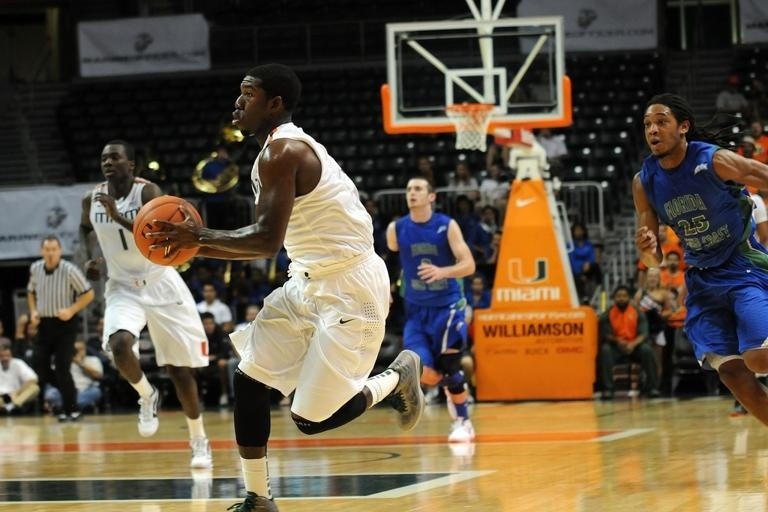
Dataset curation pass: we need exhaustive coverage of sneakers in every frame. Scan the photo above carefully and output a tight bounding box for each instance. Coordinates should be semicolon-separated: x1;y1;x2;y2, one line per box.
447;418;477;443
190;435;214;469
57;414;68;422
444;353;468;420
70;412;83;420
138;385;159;438
226;491;280;512
389;350;425;431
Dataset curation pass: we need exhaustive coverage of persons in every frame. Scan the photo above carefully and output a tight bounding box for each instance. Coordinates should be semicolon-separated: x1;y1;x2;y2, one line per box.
565;221;602;306
481;163;513;203
27;237;93;421
634;94;766;424
736;123;766;160
659;253;691;330
1;339;40;415
637;268;674;365
733;137;756;160
418;158;438;194
464;273;491;311
447;165;477;204
485;230;501;268
532;129;566;178
78;141;213;470
198;313;233;408
46;341;102;416
244;306;260;326
717;77;749;120
453;195;473;242
191;285;231;332
146;64;424;512
475;207;499;242
594;288;660;400
385;177;477;440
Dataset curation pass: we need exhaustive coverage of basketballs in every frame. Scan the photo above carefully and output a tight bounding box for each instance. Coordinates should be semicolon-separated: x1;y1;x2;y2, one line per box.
132;195;204;268
